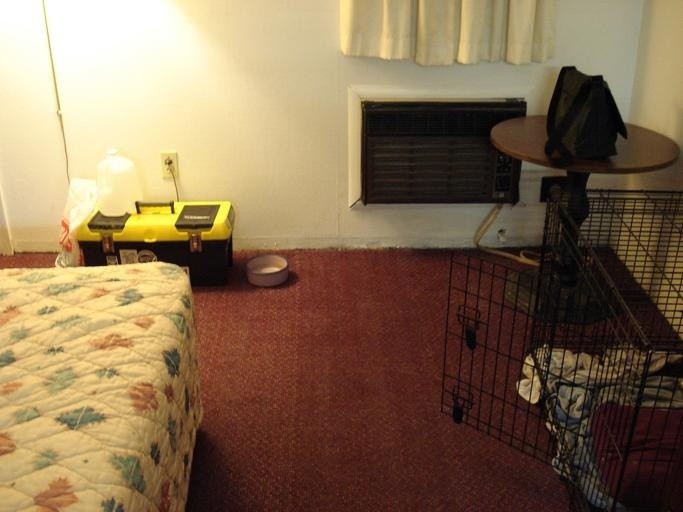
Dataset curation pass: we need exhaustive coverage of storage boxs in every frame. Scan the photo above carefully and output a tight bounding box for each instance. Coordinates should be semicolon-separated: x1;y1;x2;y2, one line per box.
74;199;235;287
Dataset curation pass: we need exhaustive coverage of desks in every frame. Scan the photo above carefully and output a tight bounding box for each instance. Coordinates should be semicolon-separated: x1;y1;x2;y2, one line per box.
488;115;682;328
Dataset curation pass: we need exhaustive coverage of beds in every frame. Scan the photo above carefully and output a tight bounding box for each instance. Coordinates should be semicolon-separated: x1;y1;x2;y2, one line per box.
1;258;200;512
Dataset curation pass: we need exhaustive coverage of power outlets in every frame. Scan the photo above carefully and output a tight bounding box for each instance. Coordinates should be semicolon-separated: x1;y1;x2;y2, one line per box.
160;152;179;178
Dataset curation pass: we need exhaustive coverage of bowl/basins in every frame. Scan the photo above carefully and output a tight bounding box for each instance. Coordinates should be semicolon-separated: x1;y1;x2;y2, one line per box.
247;256;289;286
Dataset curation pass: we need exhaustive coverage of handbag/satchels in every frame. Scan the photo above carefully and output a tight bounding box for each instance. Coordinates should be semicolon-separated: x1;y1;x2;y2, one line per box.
545;65;628;167
58;179;96;267
